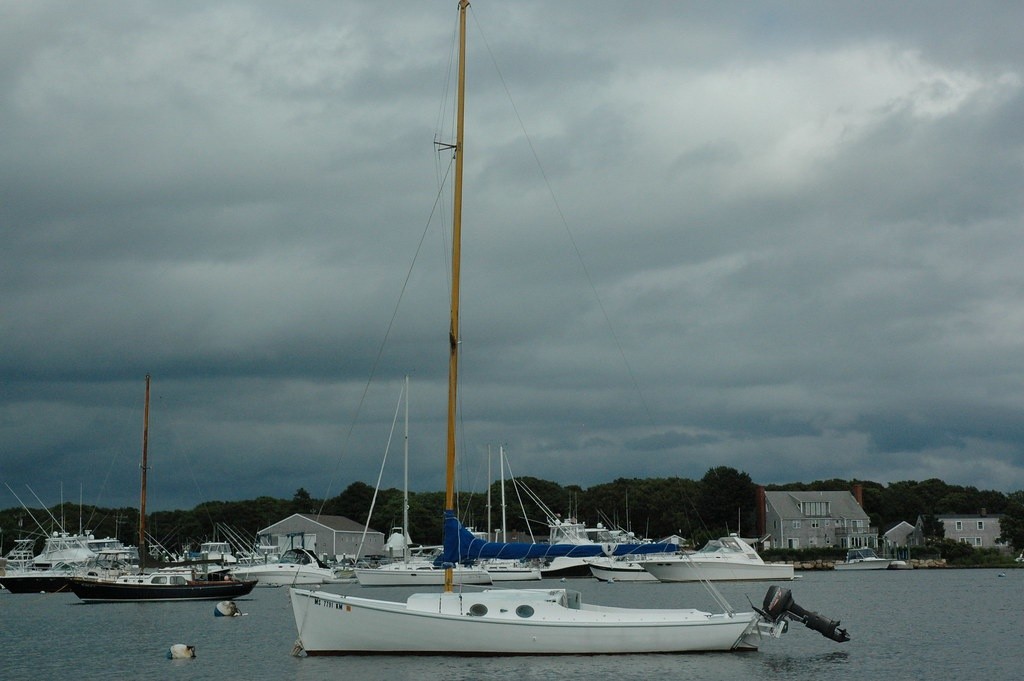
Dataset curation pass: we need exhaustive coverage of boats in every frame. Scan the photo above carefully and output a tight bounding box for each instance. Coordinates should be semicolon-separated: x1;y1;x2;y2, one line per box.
0;444;803;594
834;545;893;571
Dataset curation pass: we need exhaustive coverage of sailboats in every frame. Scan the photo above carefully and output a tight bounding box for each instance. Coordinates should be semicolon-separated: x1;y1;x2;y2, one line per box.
352;374;493;588
287;0;852;656
64;373;260;603
